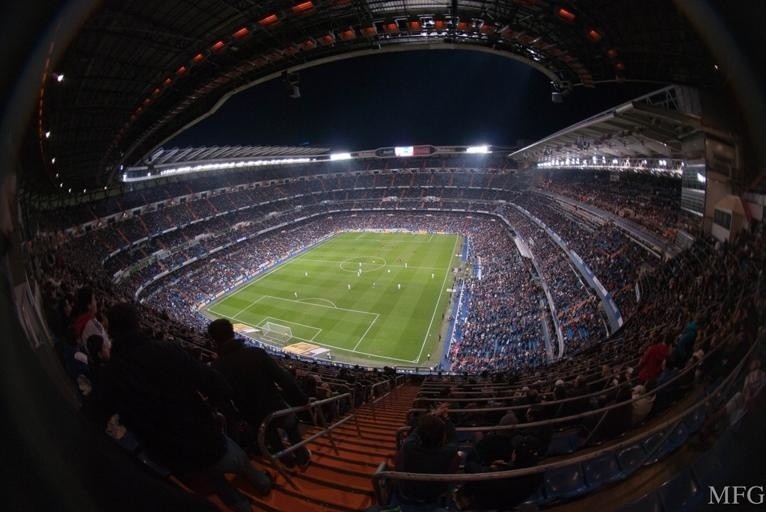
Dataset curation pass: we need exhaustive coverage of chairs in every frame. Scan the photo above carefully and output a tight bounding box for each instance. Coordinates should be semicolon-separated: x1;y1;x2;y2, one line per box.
0;229;765;512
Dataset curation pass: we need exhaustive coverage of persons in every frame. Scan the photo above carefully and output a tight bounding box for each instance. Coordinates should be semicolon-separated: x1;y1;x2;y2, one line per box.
22;158;766;512
